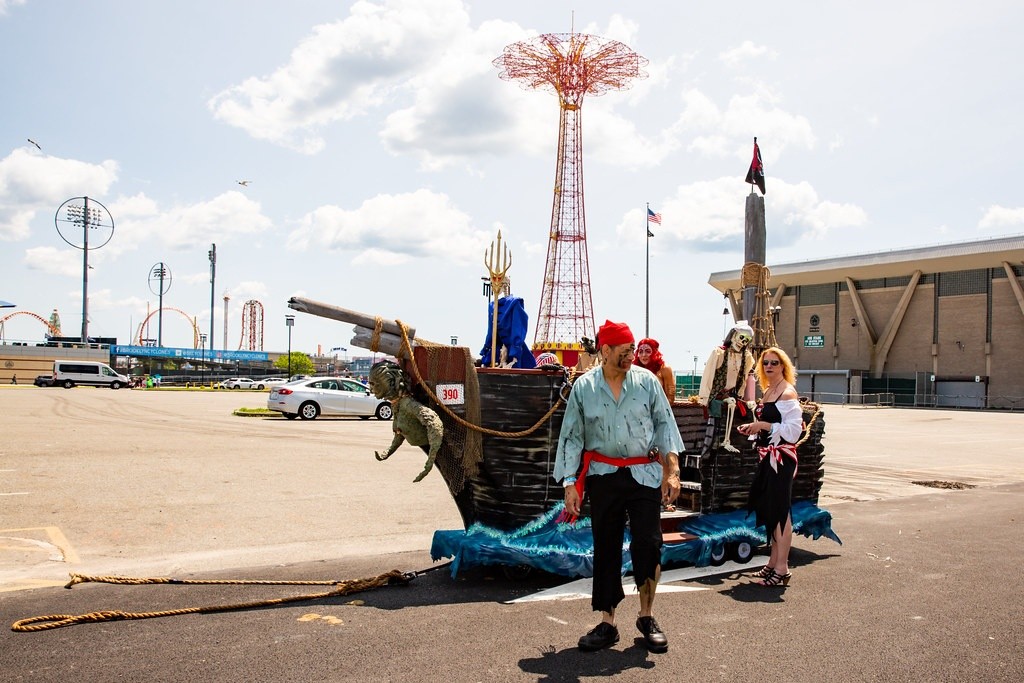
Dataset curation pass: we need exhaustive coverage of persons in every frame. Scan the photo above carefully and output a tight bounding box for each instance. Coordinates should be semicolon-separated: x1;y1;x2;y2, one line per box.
737;347;802;588
51;373;57;387
11;374;19;384
128;376;160;389
552;319;686;653
369;360;477;534
334;368;369;392
699;321;755;453
632;338;677;513
479;276;537;369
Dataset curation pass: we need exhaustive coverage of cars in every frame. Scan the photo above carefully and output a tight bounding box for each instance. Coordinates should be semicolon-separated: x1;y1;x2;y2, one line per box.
269;378;393;421
289;374;311;383
213;379;229;389
251;377;287;391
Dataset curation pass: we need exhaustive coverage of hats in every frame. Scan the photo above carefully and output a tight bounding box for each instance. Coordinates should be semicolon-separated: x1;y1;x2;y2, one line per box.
594;319;634;349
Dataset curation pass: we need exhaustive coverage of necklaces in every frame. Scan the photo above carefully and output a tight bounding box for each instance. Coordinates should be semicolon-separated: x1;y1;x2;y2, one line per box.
760;376;785;401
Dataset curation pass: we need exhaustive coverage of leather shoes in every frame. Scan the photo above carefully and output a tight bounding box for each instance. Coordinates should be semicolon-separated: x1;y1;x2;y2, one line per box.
636;615;668;649
578;622;620;649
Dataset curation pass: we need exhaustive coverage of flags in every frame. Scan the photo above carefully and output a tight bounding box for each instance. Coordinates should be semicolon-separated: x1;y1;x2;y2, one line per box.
648;230;654;238
744;142;766;195
647;208;662;225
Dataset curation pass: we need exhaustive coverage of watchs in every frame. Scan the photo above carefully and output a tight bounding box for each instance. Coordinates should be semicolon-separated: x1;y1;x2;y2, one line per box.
562;481;574;488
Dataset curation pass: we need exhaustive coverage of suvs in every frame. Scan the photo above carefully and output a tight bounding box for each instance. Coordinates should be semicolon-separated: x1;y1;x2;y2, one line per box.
53;359;129;391
34;375;64;388
227;377;255;389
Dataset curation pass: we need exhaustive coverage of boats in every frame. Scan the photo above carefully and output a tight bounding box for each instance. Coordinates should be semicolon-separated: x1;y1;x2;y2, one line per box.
285;195;828;539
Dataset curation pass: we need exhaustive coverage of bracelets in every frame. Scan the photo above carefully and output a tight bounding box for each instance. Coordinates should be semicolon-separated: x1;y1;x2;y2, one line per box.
564;476;577;482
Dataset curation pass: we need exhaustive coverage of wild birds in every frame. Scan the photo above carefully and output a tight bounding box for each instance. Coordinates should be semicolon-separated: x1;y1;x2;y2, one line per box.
87;264;95;270
26;137;42;151
234;173;253;187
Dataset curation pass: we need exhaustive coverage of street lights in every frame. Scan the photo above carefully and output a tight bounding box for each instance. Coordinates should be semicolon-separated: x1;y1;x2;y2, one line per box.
54;197;115;349
285;315;295;382
208;242;217;369
148;262;172;346
199;334;207;387
236;359;240;379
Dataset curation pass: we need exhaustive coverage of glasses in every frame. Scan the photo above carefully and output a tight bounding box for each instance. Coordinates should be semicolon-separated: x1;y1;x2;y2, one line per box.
763;359;779;366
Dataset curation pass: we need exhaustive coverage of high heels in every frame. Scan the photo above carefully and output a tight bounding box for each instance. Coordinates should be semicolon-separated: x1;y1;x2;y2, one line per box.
758;570;791;587
753;565;774;577
